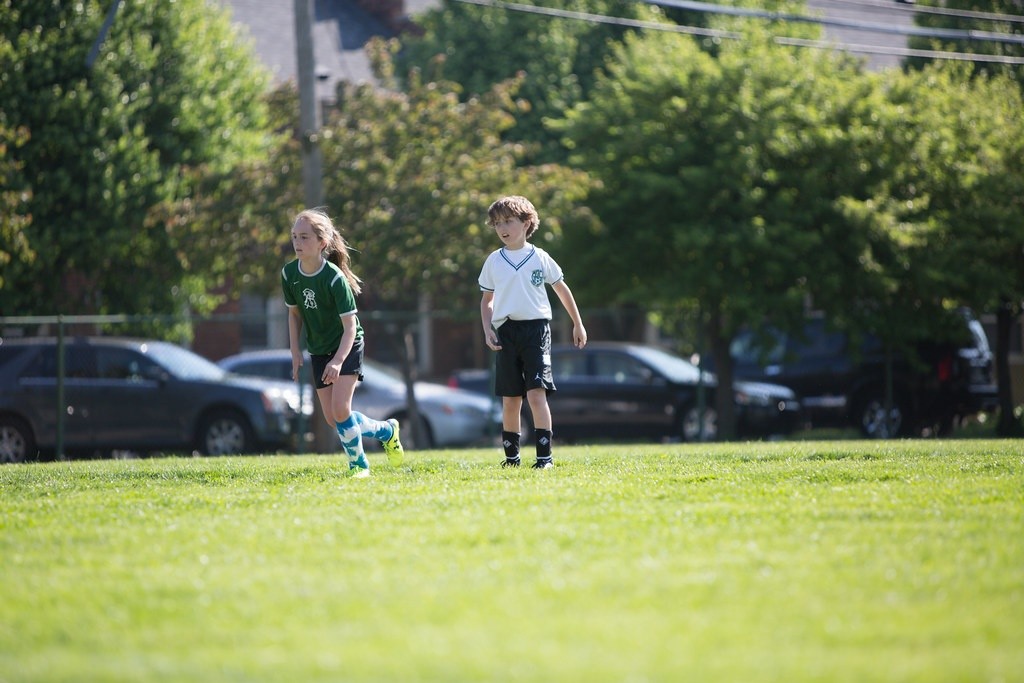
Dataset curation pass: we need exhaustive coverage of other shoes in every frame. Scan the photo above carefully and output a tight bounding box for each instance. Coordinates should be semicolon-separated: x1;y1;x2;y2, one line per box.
532;459;554;469
501;461;521;469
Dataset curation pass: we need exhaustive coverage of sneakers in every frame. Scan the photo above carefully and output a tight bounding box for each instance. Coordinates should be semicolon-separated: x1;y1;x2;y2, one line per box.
381;419;404;467
344;466;369;479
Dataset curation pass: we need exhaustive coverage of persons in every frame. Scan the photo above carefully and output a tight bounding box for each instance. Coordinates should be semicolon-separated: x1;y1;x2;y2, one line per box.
478;195;588;470
280;208;404;476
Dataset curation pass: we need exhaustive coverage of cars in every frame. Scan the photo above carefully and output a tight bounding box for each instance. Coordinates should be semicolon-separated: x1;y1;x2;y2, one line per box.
720;294;1001;437
446;340;808;439
0;335;293;458
216;348;498;452
